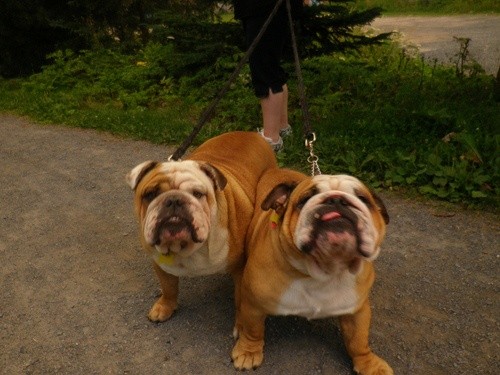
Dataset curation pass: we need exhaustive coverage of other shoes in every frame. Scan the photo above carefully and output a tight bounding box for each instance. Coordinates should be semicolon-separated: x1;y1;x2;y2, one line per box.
254;124;296;155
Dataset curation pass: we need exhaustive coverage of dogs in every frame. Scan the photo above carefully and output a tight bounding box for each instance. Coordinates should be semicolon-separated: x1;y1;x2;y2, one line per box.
230;167;394;375
124;131;278;342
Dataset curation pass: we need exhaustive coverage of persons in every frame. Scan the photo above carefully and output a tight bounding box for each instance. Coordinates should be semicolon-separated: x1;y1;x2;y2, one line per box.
233;0;312;153
